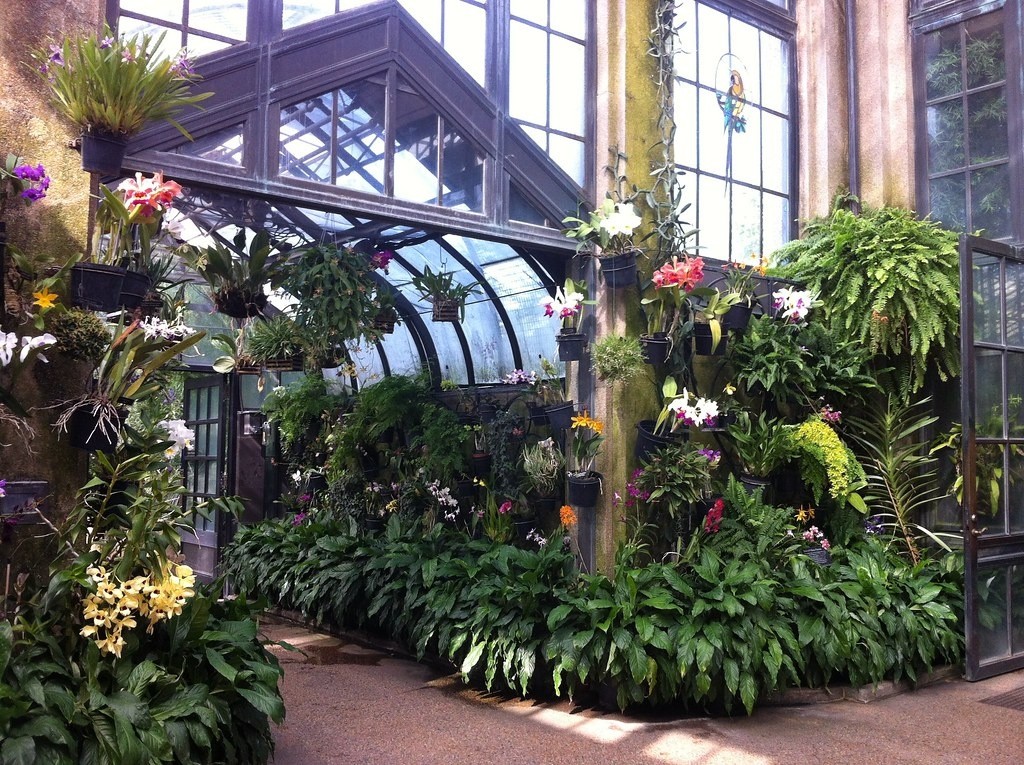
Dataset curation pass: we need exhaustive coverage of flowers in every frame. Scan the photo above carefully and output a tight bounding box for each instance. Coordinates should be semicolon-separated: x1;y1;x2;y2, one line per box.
0;0;1005;765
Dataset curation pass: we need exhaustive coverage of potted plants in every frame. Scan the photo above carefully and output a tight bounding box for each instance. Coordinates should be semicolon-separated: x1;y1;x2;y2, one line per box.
237;315;306;373
362;282;402;334
411;263;486;327
928;391;1024;575
163;227;296;319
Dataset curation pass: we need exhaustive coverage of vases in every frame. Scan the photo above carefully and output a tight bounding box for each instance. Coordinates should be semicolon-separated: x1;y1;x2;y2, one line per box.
723;305;752;330
120;270;153;309
70;262;127;312
514;519;538;541
471;453;491;474
312;348;350;369
803;546;835;569
537;480;563;503
639;333;671;365
365;519;386;538
695;322;729;356
739;475;771;504
479;404;501;422
545;400;574;430
703;411;745;428
357;444;380;481
634;419;676;463
528;406;550;427
598;251;637;288
554;333;585;361
565;470;602;507
68;407;129;451
456;479;474;498
79;130;127;175
